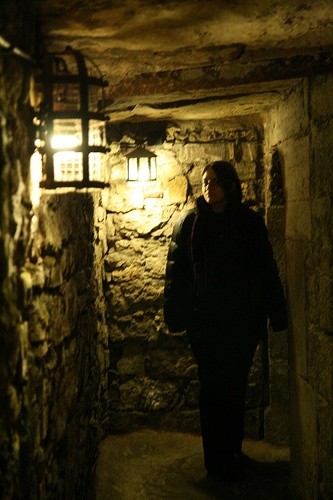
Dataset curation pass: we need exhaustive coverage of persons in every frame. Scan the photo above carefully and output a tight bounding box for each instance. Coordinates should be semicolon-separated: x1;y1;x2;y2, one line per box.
162;161;290;486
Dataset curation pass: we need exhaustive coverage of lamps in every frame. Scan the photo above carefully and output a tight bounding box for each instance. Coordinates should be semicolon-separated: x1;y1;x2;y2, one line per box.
125;132;158;182
30;45;112;190
